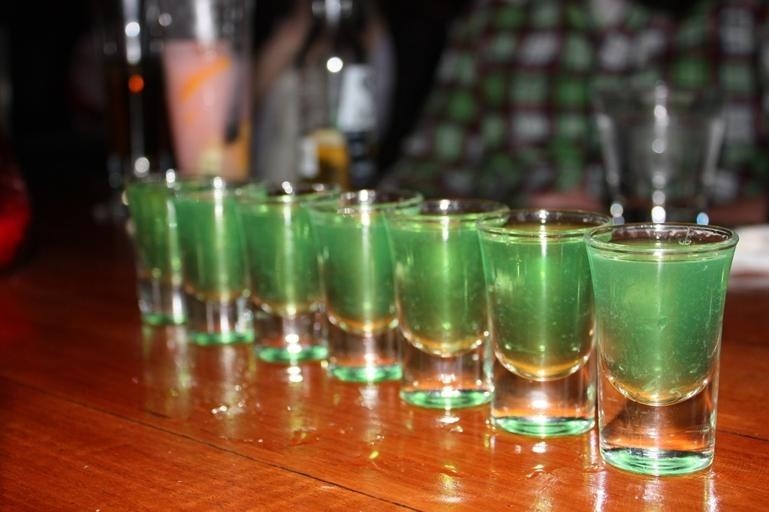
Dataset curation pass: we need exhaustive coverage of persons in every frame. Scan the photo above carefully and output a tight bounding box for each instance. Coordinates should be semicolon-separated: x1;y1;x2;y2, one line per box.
388;0;768;229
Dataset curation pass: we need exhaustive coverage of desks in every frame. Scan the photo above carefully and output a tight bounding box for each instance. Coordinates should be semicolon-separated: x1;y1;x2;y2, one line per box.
1;222;769;511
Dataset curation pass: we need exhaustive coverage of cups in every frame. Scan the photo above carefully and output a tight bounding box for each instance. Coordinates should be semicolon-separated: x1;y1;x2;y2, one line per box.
123;172;191;328
233;182;345;364
476;209;614;437
302;187;424;386
590;75;728;224
582;218;739;478
172;174;258;348
381;196;514;410
